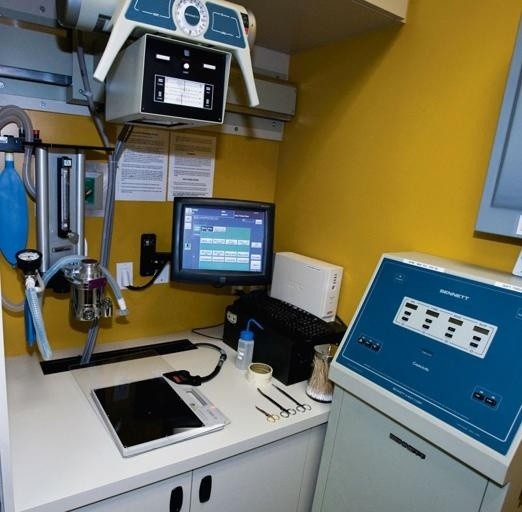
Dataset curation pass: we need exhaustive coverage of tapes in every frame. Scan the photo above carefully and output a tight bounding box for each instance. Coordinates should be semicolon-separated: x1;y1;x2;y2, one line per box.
247;363;274;384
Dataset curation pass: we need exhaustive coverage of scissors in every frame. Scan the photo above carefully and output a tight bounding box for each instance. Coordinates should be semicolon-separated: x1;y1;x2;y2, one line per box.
255;384;311;423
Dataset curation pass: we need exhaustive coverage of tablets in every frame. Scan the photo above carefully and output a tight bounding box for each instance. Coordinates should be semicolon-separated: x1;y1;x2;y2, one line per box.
91;375;231;459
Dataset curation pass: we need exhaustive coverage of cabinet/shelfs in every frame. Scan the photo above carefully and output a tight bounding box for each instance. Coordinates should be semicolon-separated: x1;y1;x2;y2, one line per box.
5;334;331;512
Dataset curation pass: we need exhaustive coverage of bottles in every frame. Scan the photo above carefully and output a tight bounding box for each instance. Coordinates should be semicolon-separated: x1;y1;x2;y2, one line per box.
236;319;263;370
305;345;339;403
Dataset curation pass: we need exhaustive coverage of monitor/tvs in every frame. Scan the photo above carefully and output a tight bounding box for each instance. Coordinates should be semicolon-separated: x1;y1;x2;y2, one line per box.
170;196;276;287
